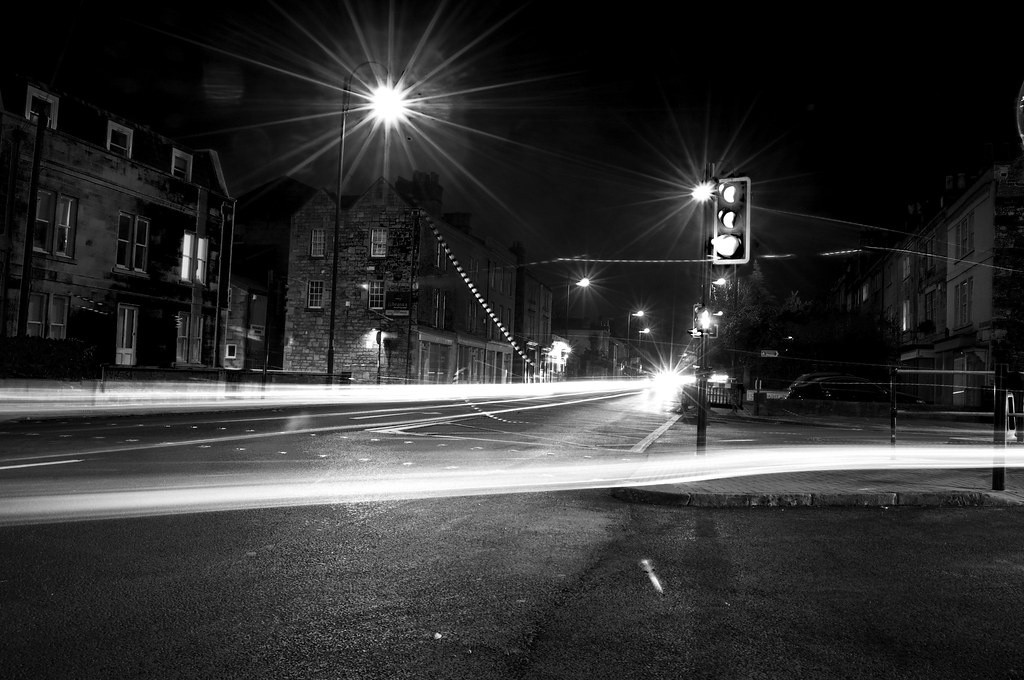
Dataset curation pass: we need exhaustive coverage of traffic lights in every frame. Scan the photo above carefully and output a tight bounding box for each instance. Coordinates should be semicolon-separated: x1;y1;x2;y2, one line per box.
712;176;752;267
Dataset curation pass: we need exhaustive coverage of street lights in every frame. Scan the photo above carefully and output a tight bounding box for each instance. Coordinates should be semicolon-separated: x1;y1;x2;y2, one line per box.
626;310;644;377
565;274;589;380
324;59;401;389
636;328;651;376
